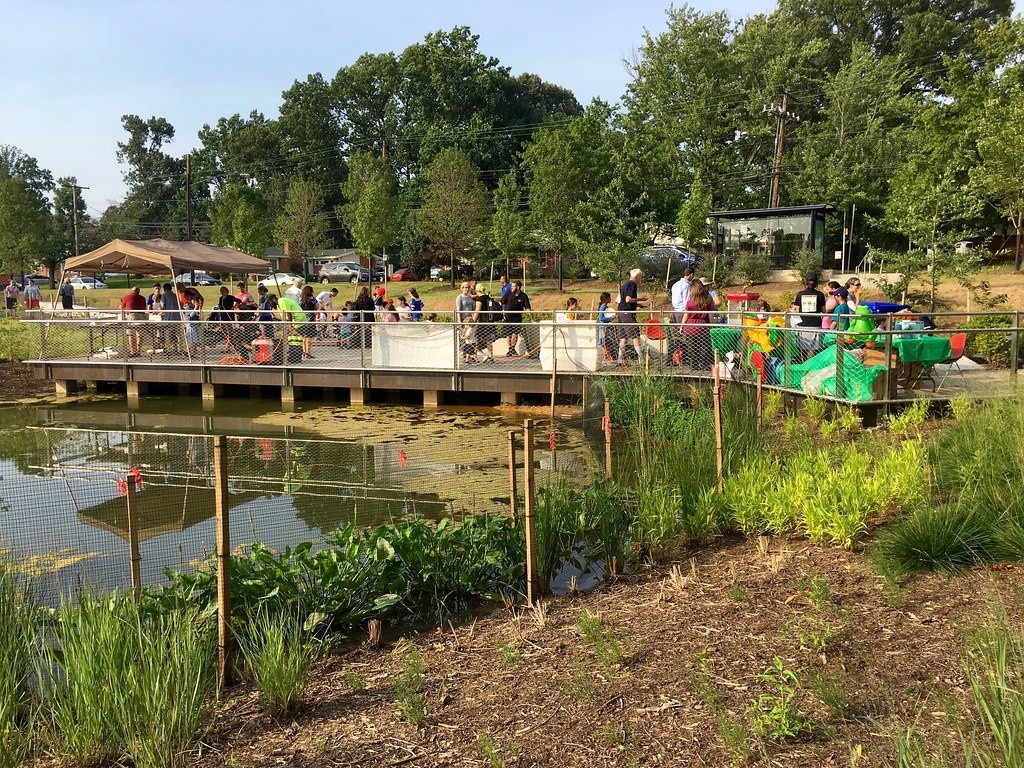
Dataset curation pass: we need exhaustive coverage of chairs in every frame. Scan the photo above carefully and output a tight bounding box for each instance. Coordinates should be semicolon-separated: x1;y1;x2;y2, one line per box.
911;333;969;393
646;318;667;367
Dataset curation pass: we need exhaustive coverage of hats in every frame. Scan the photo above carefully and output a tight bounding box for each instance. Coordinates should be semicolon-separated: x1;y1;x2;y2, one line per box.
264;294;276;303
189;295;195;301
386;298;394;304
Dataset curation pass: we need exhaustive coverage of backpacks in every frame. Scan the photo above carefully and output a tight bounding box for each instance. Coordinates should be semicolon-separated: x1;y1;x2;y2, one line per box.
487;298;502;321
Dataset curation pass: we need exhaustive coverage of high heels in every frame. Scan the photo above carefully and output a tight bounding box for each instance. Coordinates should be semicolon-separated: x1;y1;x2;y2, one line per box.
303;352;312;359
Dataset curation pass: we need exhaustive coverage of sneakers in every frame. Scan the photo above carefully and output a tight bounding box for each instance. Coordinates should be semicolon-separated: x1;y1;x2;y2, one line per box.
483;358;494;364
507;349;518;357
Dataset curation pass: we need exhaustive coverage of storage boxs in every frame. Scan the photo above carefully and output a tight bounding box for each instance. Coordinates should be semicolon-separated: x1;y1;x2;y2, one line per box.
893;321;924;339
863;347;922;390
870;368;898;400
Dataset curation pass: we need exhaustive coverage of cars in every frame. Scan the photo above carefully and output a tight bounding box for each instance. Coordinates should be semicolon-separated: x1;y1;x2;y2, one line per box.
390;268;418;282
258;273;306;285
317;261;385;284
24;273;59;285
170;272;222;286
69;277;108;289
638;245;705;278
429;263;464;280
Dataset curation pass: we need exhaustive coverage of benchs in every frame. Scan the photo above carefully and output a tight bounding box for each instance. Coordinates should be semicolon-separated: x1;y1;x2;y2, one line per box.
538;319;604;372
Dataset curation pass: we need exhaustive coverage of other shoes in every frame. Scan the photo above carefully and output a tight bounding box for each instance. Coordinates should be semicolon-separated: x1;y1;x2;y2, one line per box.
187;355;194;358
347;345;350;349
476;361;479;366
128;354;141;357
460;357;464;363
338;347;342;350
466;362;468;365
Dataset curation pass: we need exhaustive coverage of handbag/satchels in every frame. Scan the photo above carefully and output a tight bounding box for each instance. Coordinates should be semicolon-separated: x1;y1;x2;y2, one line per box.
59;288;65;295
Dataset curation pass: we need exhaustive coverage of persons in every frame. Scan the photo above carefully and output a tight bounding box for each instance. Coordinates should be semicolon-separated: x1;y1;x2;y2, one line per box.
615;268;648;367
758;272;863;364
669;267;722;371
457;275;536;366
59;274;75;319
23;279;42;311
124;434;312;495
564;297;581;320
5;279;20;316
597;292;617;362
120;277;425;363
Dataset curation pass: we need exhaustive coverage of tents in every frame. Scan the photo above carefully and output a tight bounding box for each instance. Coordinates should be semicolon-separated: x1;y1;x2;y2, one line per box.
42;430;267;543
40;239;283;363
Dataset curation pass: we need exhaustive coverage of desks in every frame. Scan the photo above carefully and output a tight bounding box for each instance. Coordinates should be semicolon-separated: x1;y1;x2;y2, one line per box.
822;334;952;389
79;323;175;363
709;327;798;362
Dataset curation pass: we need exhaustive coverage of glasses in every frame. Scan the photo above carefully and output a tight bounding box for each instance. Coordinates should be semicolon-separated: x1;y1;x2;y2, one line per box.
155;288;159;289
